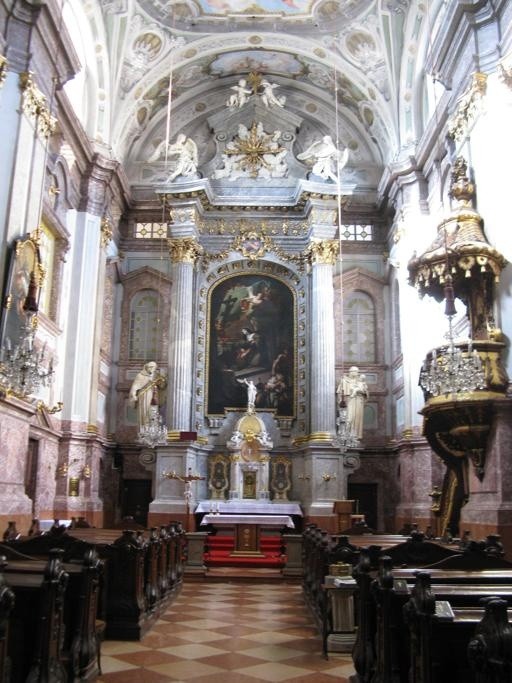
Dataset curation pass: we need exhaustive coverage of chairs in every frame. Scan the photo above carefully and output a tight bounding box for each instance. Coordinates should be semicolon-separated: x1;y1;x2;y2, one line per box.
2;518;188;683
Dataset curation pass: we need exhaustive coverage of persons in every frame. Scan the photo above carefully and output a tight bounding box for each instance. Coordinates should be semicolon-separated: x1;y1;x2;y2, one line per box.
130;360;157;432
230;75;252;109
243;379;258;409
166;133;198;184
260;80;286;109
215;282;272;370
337;366;368;440
297;136;350;184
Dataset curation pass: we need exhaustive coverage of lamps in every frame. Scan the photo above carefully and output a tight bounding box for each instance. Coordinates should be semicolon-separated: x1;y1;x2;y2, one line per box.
3;0;66;388
330;54;359;453
420;0;486;395
132;10;177;450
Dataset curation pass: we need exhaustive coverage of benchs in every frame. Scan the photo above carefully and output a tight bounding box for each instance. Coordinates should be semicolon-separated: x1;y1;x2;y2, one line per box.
304;525;512;683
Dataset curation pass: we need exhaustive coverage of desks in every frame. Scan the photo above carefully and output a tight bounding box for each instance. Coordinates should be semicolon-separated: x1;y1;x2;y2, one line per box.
195;502;304;519
201;515;293;577
